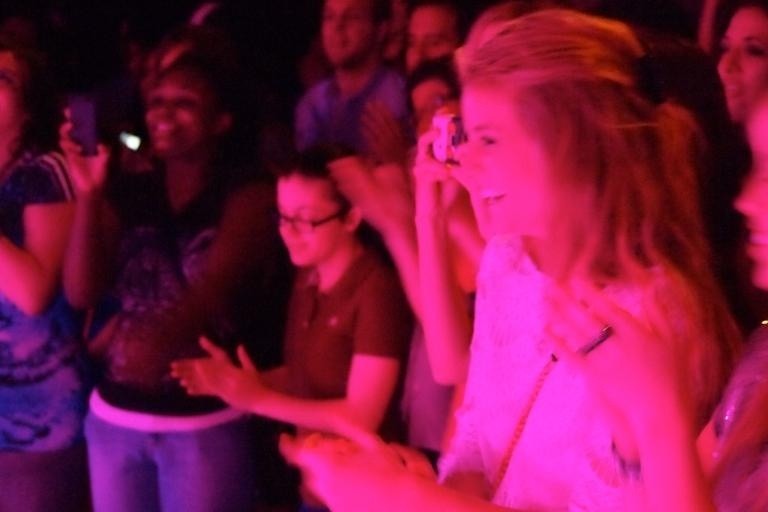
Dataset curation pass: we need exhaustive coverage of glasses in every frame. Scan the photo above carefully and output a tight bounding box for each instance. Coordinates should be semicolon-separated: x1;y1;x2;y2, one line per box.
273;209;346;234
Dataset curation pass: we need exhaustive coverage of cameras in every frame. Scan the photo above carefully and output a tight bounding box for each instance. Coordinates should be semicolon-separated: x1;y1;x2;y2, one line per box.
430;113;466;165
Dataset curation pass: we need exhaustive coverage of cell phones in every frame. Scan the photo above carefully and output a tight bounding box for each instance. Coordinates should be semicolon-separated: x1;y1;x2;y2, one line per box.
68;97;98;155
118;131;142;152
